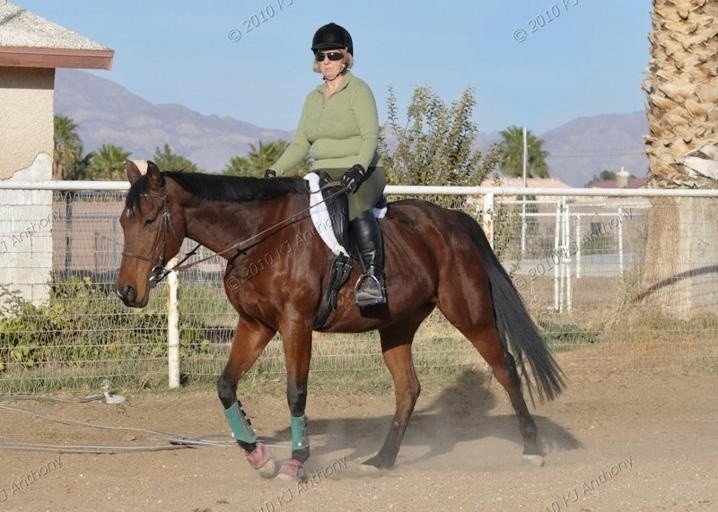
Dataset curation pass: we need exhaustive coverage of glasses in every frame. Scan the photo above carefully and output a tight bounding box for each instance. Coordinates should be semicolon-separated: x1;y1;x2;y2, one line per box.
314;52;343;61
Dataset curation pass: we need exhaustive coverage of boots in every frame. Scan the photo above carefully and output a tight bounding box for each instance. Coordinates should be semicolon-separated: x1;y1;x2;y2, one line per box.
349;209;385;300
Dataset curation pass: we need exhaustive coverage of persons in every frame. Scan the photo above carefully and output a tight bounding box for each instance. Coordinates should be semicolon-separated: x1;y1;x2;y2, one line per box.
264;22;385;299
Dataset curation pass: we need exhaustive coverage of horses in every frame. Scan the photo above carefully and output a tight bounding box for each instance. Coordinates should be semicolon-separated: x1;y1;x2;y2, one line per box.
115;160;568;486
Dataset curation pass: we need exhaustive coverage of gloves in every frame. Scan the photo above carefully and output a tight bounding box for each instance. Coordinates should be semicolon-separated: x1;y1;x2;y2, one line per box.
340;164;366;193
264;169;275;177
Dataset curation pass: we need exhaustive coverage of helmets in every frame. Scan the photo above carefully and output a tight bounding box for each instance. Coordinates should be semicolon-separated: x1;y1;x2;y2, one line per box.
312;23;353;56
